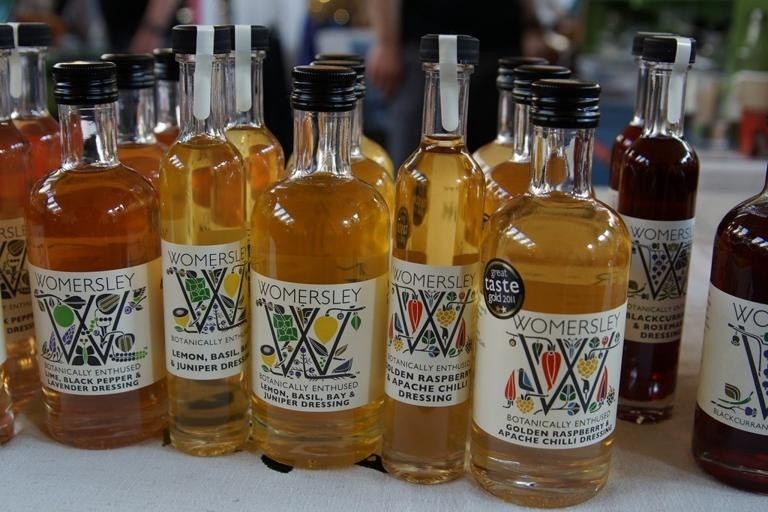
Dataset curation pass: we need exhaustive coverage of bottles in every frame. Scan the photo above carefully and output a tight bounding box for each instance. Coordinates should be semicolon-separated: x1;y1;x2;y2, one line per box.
101;51;164;188
469;78;630;510
470;57;547;176
25;61;169;450
378;32;487;486
283;59;390;215
480;64;571;232
692;165;768;490
250;63;390;470
0;23;41;415
11;21;66;187
285;54;394;180
157;24;249;458
616;36;698;424
609;31;673;213
153;47;180;151
211;25;284;239
